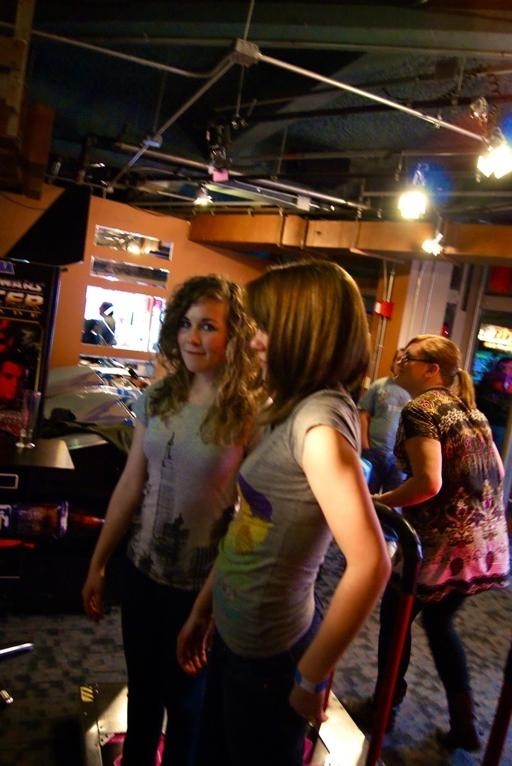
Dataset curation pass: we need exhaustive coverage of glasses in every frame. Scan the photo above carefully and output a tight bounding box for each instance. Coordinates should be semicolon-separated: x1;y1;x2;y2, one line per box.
402;354;433;364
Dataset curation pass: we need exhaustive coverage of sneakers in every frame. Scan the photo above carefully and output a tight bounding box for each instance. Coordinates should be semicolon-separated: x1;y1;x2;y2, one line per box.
435;726;482;752
342;699;397;734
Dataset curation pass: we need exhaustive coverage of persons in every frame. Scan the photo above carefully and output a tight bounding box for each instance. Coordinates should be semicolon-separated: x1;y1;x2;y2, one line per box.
344;334;512;751
81;274;257;766
357;347;410;493
95;298;117;343
0;357;31;403
176;260;392;765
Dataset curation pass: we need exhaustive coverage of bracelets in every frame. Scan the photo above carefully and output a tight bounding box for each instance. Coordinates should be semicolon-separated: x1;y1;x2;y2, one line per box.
291;669;330;695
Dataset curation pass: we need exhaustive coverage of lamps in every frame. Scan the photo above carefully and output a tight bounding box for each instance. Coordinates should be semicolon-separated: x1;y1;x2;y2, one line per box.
192;181;211;208
469;95;512;179
397;164;434;217
206;125;230;183
